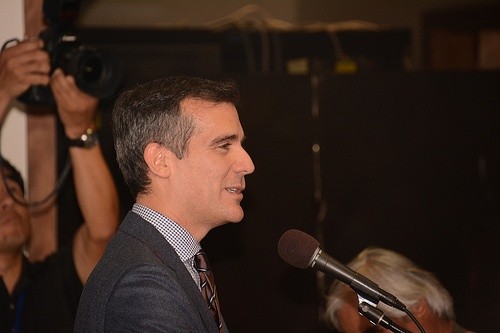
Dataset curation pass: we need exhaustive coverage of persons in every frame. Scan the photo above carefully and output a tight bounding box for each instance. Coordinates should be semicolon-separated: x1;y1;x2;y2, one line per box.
0;37;120;333
326;246;472;333
71;77;255;333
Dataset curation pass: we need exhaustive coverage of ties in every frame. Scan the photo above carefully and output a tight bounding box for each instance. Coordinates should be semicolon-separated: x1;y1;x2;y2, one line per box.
195;248;230;333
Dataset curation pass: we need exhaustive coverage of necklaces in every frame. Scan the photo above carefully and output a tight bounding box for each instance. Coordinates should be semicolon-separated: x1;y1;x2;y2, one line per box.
450;320;454;333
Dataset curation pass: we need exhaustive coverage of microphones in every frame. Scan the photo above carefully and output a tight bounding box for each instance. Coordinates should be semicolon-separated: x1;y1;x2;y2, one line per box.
278;229;407;310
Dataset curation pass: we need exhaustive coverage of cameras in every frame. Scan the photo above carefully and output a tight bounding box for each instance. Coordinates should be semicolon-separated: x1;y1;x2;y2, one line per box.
17;18;131;105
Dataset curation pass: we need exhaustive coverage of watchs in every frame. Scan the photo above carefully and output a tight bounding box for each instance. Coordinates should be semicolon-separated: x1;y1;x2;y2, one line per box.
66;129;98;149
